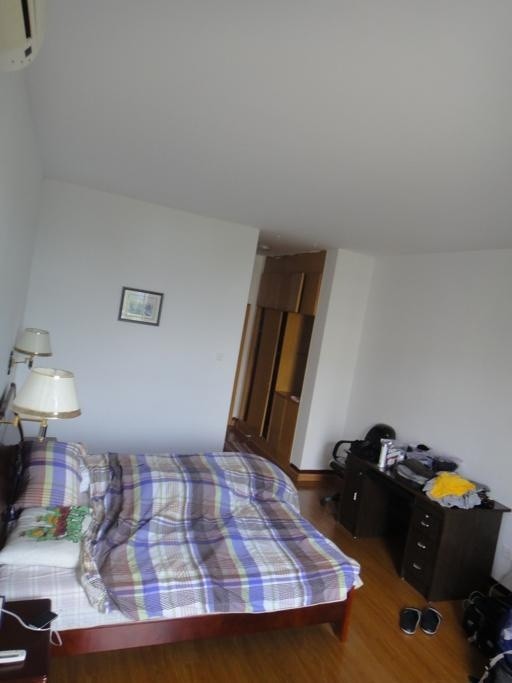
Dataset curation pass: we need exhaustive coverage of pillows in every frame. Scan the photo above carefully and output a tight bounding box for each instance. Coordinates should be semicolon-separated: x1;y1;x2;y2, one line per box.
0;505;95;570
8;440;88;518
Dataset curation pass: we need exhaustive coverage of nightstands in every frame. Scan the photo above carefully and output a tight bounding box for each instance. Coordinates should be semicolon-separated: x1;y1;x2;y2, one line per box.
0;596;52;681
23;435;58;442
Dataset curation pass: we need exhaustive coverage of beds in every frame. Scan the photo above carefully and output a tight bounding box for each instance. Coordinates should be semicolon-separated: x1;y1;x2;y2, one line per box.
0;382;365;656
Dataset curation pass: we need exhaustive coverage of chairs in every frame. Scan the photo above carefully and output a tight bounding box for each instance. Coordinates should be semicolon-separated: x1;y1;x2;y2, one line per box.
318;421;396;504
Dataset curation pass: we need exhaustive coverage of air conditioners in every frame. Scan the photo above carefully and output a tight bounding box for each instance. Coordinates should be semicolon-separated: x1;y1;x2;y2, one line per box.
1;0;47;73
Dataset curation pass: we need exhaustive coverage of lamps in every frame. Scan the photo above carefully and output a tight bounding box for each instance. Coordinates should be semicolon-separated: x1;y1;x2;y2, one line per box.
0;366;83;443
7;326;54;374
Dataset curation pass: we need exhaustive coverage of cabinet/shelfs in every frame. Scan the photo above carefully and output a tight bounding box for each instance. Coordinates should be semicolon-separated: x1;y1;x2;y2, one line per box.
256;271;306;312
266;390;300;459
244;306;305;439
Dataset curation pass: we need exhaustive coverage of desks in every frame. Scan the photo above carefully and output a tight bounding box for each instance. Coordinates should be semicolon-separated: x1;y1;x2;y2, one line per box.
337;448;512;604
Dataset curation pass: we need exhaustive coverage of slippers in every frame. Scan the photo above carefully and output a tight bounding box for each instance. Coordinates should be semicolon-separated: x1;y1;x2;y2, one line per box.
419;606;443;635
399;606;421;635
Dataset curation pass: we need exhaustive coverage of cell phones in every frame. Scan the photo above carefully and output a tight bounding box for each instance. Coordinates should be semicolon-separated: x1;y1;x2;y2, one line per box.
27;610;59;631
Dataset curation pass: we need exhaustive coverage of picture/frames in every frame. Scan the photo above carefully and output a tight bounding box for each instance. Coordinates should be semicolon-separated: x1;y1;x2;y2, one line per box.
117;286;163;326
